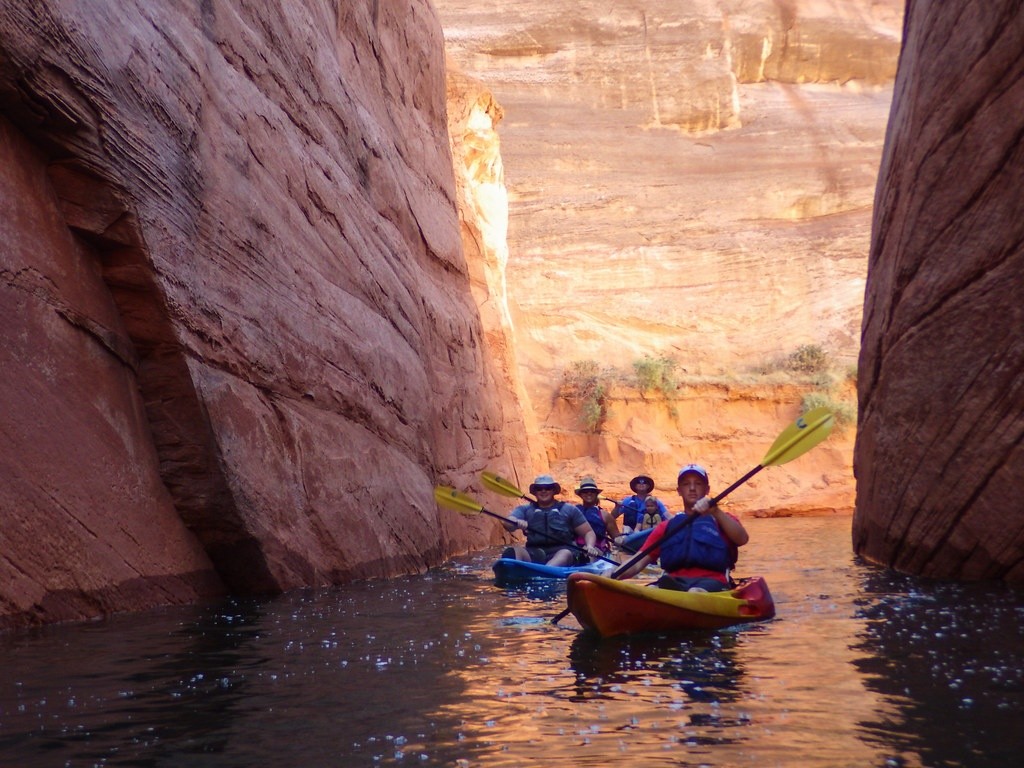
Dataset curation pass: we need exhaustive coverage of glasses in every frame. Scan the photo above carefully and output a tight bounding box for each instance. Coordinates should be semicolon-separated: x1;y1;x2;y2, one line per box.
636;480;648;485
579;489;596;493
533;485;554;491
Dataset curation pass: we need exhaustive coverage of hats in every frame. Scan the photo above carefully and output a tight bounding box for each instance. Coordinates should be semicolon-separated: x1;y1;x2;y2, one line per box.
630;474;655;494
677;464;709;484
529;475;561;496
574;478;603;497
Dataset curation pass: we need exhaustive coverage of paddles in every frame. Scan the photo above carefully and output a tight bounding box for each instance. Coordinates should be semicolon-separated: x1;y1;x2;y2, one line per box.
479;470;660;566
595;494;645;515
433;484;622;567
541;406;834;626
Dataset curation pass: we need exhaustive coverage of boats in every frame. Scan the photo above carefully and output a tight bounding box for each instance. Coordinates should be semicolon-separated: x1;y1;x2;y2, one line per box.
619;527;657;558
566;571;778;642
491;554;614;588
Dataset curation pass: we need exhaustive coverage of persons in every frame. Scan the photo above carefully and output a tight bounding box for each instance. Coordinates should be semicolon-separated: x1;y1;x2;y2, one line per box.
634;495;662;532
573;476;621;562
608;473;672;540
600;463;749;594
501;475;598;569
